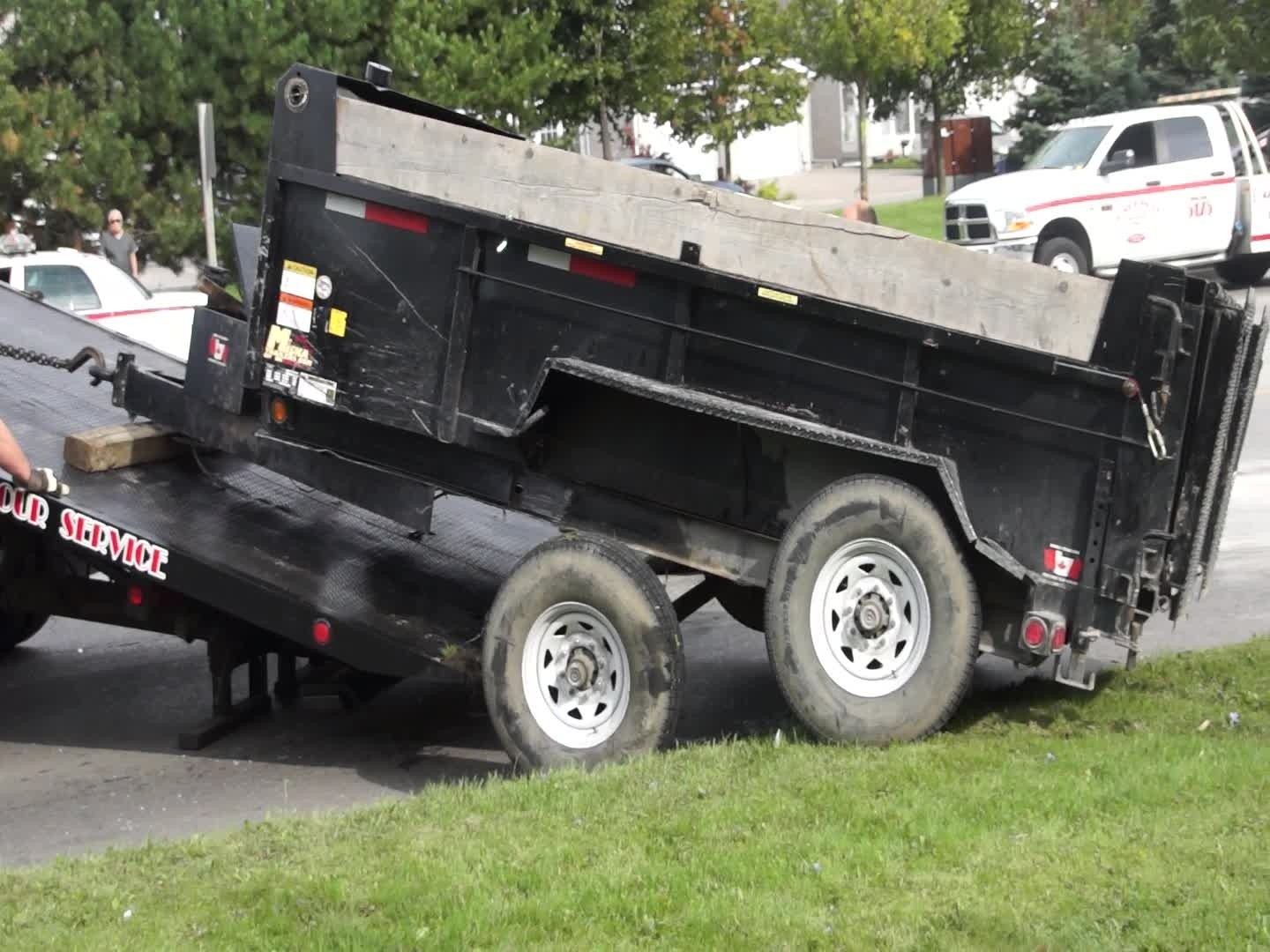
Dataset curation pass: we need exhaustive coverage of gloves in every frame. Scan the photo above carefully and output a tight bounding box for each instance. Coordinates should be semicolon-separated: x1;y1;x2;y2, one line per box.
12;467;70;498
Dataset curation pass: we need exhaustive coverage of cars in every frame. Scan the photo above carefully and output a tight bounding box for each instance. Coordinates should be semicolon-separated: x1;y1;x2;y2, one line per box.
609;155;747;194
0;232;211;363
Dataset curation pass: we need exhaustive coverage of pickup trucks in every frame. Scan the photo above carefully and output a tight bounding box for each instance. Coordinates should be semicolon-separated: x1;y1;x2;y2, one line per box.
939;82;1270;286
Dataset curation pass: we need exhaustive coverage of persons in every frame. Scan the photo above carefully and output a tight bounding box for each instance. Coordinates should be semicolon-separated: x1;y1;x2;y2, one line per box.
0;411;71;498
846;201;877;225
100;207;137;285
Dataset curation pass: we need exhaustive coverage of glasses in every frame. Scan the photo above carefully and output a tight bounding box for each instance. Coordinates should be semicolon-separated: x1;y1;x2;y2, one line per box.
110;219;121;224
